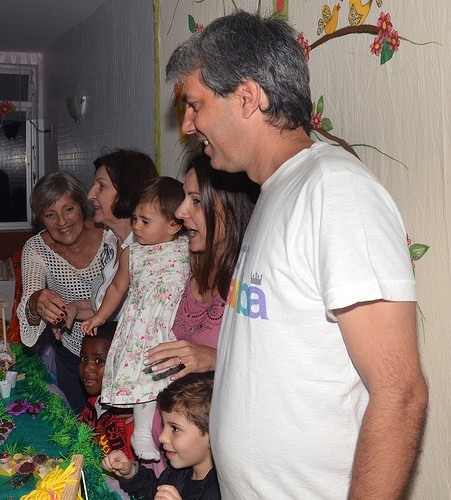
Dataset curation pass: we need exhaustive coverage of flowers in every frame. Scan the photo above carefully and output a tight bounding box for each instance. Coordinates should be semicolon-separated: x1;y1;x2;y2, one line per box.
6;399;45;416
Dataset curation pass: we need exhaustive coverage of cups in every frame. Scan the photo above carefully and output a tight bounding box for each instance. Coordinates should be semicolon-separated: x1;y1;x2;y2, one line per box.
0;381;12;398
6;370;17;389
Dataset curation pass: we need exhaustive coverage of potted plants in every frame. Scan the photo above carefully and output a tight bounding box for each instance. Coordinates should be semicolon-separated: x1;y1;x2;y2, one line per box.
0;351;18;399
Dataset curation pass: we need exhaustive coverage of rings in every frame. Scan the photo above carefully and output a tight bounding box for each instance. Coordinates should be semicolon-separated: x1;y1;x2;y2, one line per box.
41;307;45;312
179;358;182;365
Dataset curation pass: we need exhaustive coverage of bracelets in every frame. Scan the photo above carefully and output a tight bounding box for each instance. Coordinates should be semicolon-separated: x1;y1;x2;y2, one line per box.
26;300;40;321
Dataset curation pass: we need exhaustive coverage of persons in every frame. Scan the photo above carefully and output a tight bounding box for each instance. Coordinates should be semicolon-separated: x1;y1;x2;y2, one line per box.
116;151;263;478
51;147;159;341
16;171;108;414
81;176;192;462
101;370;222;500
167;8;429;500
79;321;138;465
